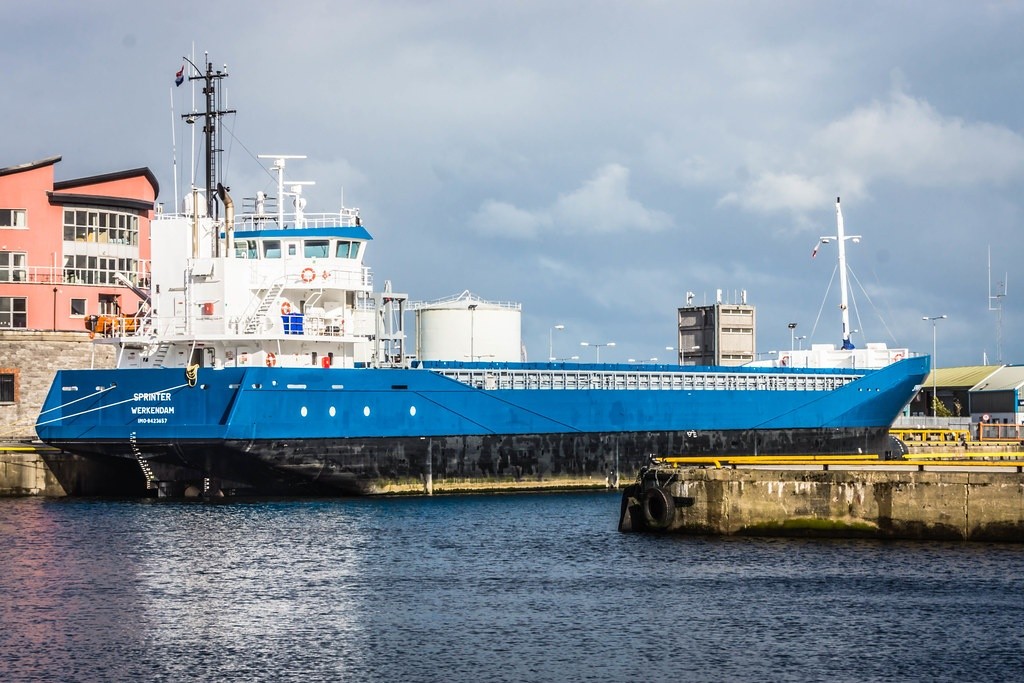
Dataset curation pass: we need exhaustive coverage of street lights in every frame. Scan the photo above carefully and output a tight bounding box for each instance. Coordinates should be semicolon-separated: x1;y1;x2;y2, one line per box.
850;329;858;345
468;305;477;361
665;346;701;365
788;323;798;351
549;325;564;363
794;336;806;351
580;342;616;364
464;354;496;361
922;314;947;430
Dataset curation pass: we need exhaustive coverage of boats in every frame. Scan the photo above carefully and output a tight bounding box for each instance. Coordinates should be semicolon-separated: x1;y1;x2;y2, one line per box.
34;38;935;502
84;313;148;333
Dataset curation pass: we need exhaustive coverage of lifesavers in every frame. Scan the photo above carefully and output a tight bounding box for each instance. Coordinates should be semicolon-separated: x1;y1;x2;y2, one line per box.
894;354;904;362
266;352;277;367
641;486;676;530
281;302;291;315
781;356;789;366
301;268;316;282
